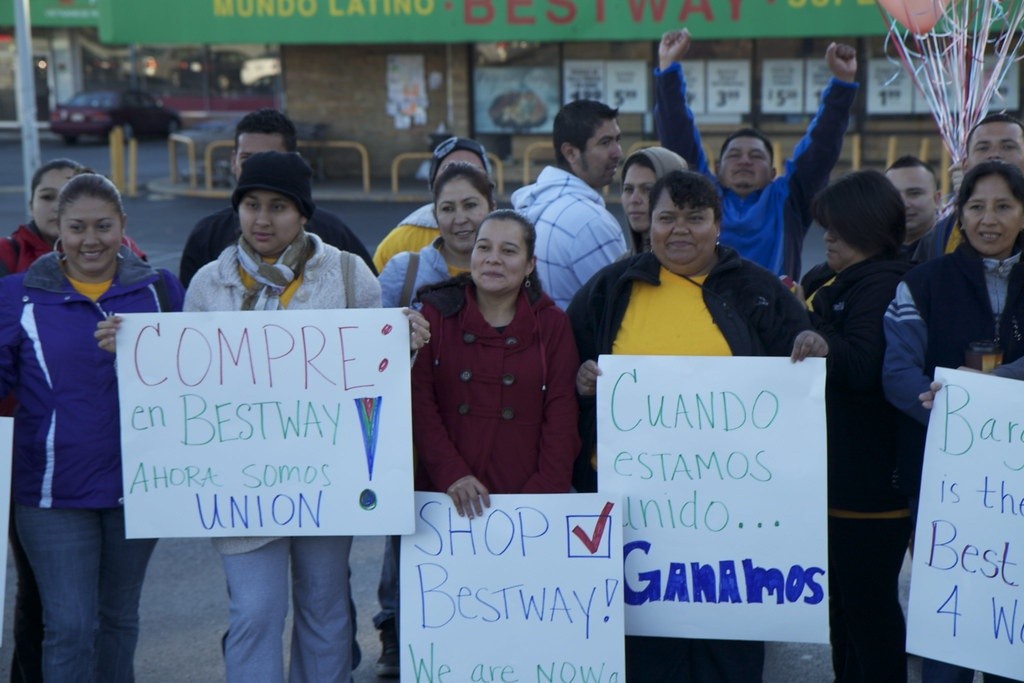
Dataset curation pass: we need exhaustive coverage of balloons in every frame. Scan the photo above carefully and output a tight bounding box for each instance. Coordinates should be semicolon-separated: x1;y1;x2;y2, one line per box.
879;0;951;38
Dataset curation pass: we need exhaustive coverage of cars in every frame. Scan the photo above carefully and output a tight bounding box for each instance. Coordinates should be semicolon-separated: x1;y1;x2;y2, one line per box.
49;88;182;144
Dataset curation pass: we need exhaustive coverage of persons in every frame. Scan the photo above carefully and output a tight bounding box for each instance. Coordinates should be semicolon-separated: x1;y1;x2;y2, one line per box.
655;26;859;286
0;157;148;683
889;118;1024;249
180;108;380;683
567;171;830;683
510;99;688;308
882;159;1024;683
373;137;515;683
782;168;923;683
0;173;187;683
94;146;432;683
412;210;579;520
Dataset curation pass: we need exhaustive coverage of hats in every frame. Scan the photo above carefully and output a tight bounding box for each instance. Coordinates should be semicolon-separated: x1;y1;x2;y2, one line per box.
230;150;314;220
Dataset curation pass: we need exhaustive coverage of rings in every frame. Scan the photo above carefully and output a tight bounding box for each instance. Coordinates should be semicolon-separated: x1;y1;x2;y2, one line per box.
424;338;430;343
